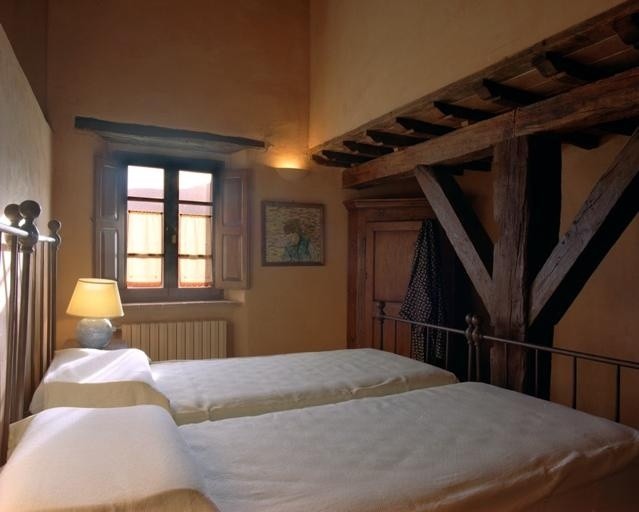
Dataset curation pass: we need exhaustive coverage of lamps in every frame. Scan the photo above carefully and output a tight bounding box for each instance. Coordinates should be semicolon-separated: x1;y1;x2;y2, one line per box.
276;164;313;186
63;276;126;350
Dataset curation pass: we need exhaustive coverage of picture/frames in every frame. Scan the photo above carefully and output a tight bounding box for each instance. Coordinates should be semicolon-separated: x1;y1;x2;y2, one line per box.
260;200;327;268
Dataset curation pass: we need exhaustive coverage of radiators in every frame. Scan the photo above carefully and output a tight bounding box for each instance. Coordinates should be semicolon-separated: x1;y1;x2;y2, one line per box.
113;320;228;361
